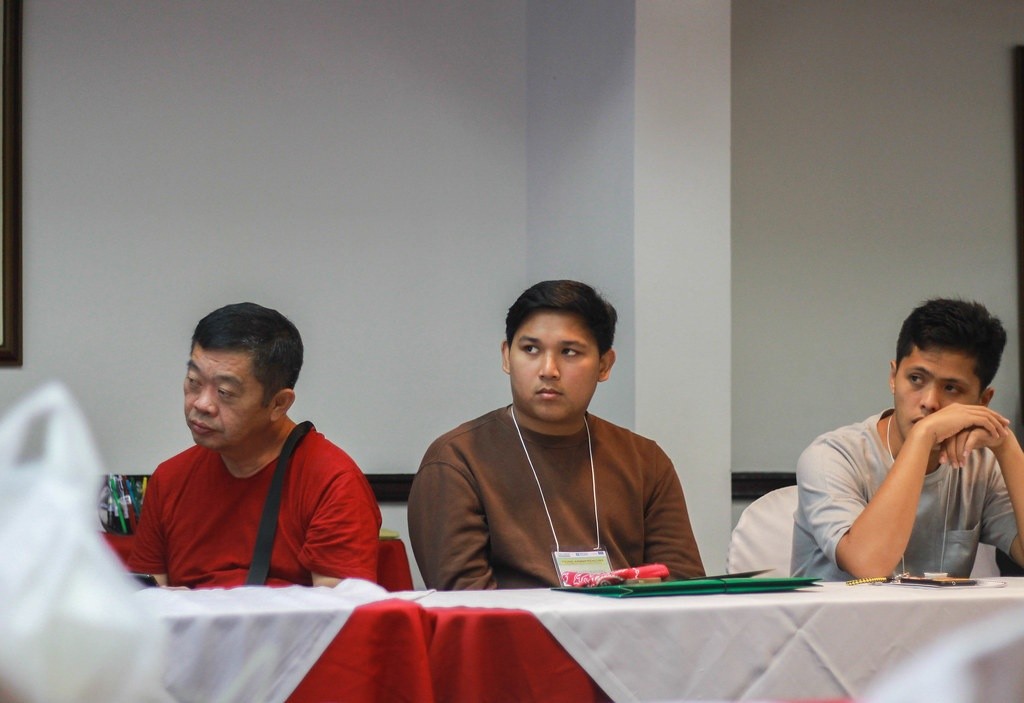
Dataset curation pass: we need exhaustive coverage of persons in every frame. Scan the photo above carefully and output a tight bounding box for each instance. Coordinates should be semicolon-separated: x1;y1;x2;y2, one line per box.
125;302;383;591
790;297;1024;582
407;281;708;591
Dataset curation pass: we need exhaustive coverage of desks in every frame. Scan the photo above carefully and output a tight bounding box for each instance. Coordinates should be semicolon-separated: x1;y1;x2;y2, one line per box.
143;574;1024;703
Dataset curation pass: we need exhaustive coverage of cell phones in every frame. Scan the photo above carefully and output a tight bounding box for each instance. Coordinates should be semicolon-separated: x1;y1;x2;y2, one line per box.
901;575;976;587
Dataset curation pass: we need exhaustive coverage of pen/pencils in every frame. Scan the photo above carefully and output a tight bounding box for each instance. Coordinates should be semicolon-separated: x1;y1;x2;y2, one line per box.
106;474;148;532
